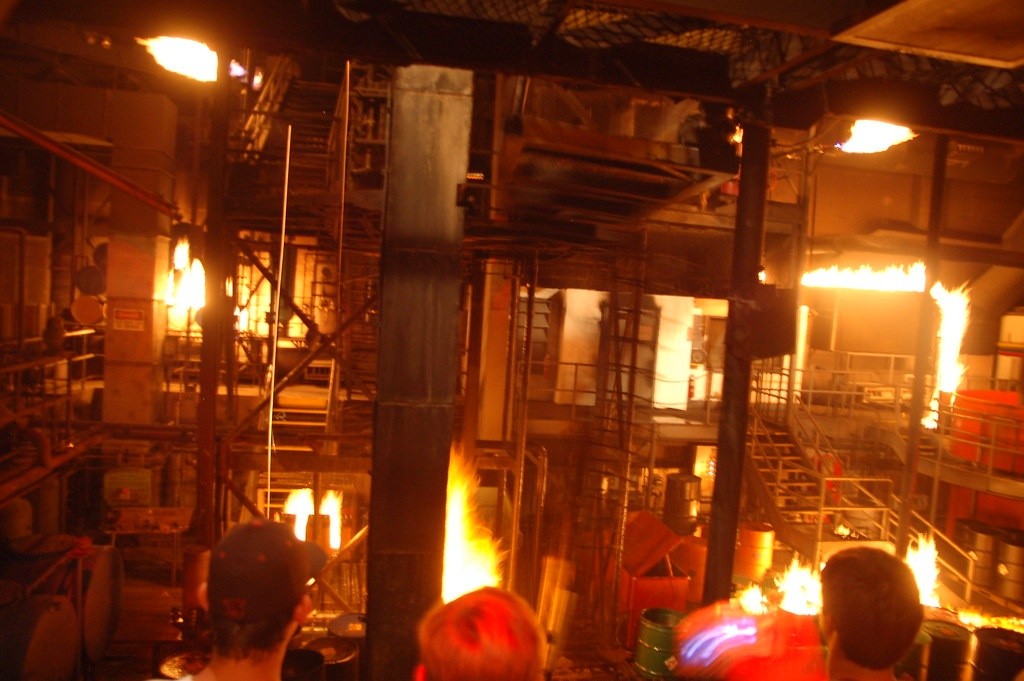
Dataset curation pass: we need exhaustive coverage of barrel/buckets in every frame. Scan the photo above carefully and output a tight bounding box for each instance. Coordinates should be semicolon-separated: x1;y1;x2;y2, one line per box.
969;627;1024;681
843;498;879;529
954;518;1024;605
282;613;366;681
160;650;211;679
634;607;688;681
663;473;702;536
739;523;774;583
893;628;932;681
921;619;972;681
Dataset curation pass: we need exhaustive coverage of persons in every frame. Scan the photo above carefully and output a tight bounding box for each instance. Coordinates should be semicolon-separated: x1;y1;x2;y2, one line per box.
819;546;922;681
412;585;549;681
179;517;313;681
